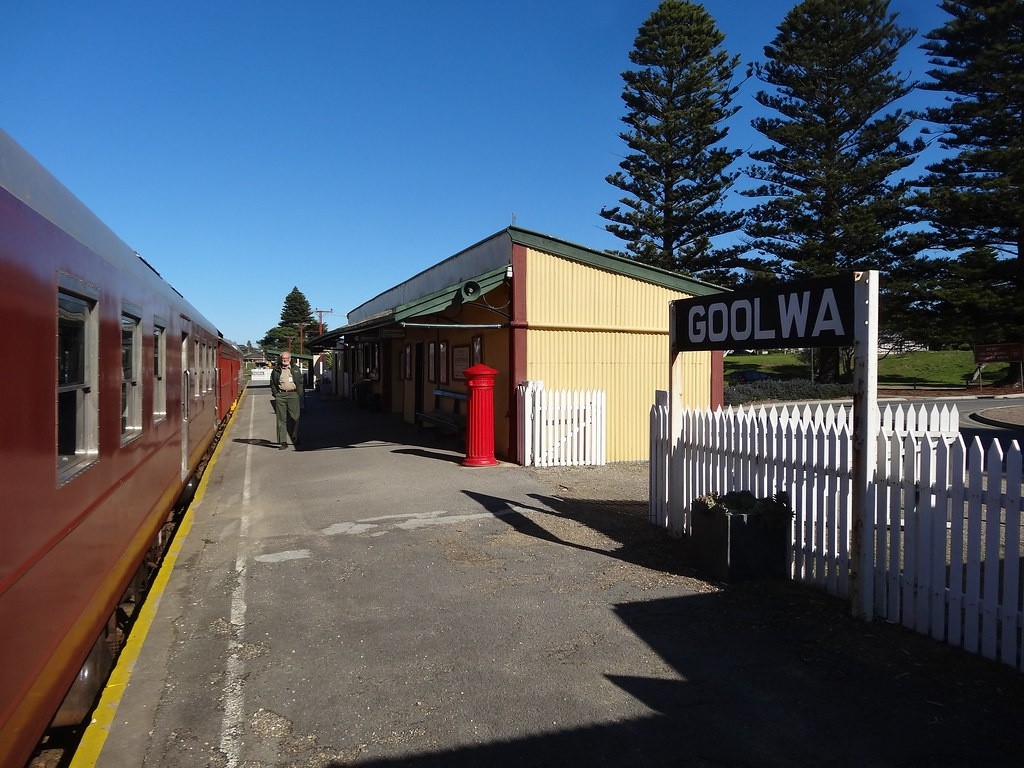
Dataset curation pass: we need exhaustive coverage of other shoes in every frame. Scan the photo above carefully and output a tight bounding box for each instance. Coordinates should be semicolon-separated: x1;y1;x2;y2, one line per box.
280;443;288;450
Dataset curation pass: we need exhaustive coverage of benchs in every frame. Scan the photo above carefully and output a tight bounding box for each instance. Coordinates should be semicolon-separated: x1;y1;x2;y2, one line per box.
414;389;469;449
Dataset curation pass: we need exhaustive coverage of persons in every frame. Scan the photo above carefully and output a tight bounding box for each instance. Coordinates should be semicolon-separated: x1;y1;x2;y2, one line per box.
269;351;304;451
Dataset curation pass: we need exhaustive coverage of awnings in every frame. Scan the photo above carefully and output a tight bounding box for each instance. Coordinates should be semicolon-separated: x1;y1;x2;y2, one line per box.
266;350;313;360
303;264;513;352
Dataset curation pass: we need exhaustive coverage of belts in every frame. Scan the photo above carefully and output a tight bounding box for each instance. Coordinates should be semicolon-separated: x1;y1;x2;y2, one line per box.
280;389;297;392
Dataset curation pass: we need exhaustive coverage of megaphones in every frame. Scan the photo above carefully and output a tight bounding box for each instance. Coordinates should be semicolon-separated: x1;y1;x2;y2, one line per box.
461;280;481;301
